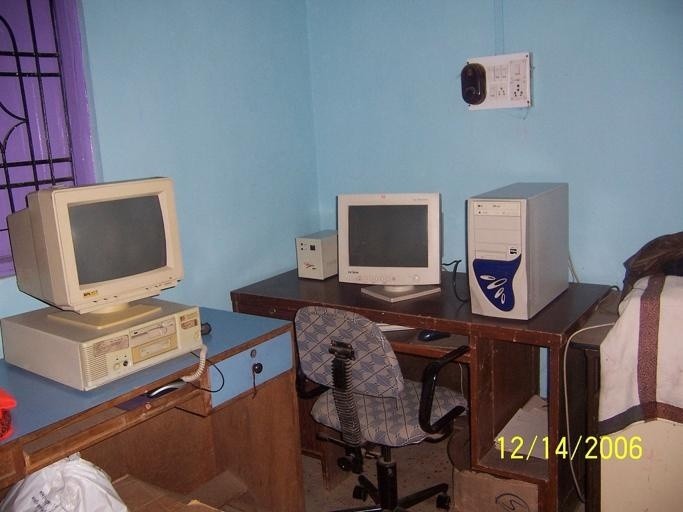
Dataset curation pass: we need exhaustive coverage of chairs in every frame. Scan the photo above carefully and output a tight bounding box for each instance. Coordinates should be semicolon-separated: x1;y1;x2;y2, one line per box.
291;306;470;512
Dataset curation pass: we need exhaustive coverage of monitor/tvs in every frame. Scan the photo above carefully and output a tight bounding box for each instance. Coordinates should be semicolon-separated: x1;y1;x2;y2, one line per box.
335;191;443;302
7;176;185;330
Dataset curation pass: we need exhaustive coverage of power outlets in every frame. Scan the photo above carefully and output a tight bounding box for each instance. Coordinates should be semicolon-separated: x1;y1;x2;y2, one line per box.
466;51;533;110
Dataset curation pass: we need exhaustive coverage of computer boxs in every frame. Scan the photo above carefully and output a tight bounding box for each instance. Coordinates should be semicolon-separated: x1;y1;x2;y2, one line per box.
465;181;572;321
0;298;204;391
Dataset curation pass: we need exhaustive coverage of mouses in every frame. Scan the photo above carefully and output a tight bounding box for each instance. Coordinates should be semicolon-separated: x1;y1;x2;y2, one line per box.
419;331;450;342
148;380;186;399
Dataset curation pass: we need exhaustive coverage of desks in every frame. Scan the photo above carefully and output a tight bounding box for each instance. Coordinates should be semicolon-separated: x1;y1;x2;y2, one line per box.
231;269;612;512
570;291;622;512
0;305;304;512
